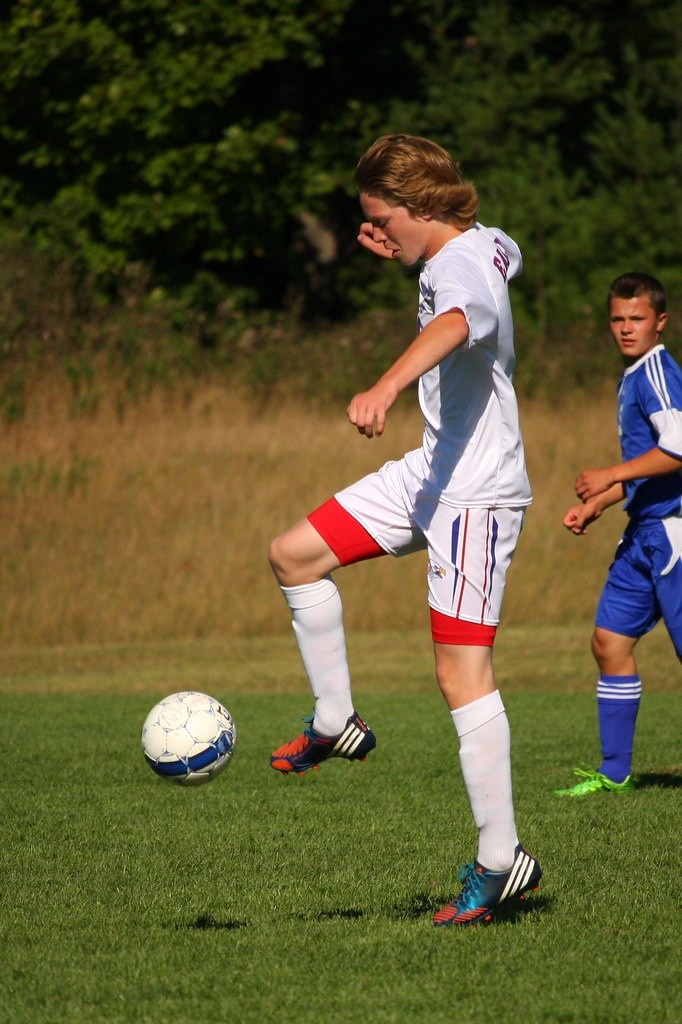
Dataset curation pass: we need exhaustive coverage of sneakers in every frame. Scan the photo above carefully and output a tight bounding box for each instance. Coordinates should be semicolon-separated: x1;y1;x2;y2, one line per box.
433;845;543;928
271;712;376;778
552;767;634;797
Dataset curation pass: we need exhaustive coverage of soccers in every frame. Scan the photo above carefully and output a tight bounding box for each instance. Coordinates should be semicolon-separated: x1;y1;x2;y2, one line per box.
141;690;237;787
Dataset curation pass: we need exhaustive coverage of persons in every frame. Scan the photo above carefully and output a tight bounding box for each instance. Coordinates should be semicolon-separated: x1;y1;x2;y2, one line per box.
266;135;544;922
552;274;682;797
